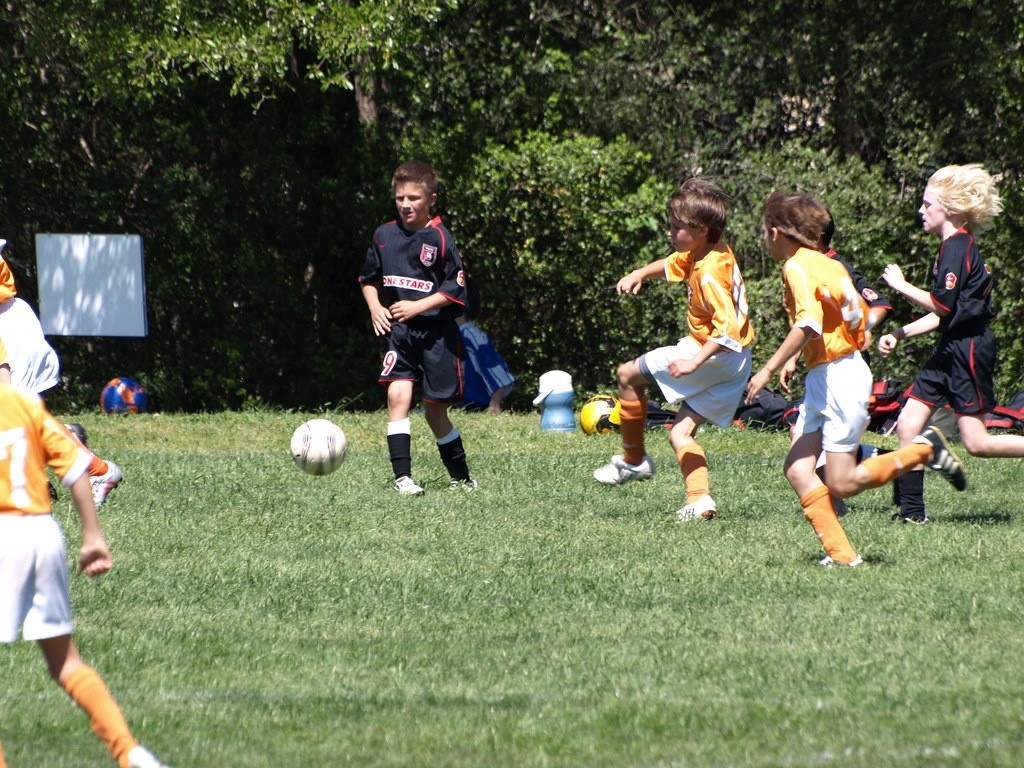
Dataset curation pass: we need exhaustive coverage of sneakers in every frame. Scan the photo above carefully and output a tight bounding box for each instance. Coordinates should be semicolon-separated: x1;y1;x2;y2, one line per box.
676;495;717;523
913;426;967;493
89;460;123;507
450;480;477;492
593;455;654;485
821;557;863;567
393;476;424;495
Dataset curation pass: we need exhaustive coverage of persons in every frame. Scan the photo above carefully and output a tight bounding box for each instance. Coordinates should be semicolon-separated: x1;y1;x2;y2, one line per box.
0;384;159;768
814;210;897;518
594;179;755;522
745;192;967;567
878;164;1024;522
455;316;516;411
0;253;124;505
358;160;476;496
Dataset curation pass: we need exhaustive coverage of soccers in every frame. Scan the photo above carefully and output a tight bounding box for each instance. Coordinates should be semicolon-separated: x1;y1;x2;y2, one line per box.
580;394;622;436
100;378;148;415
290;418;347;476
